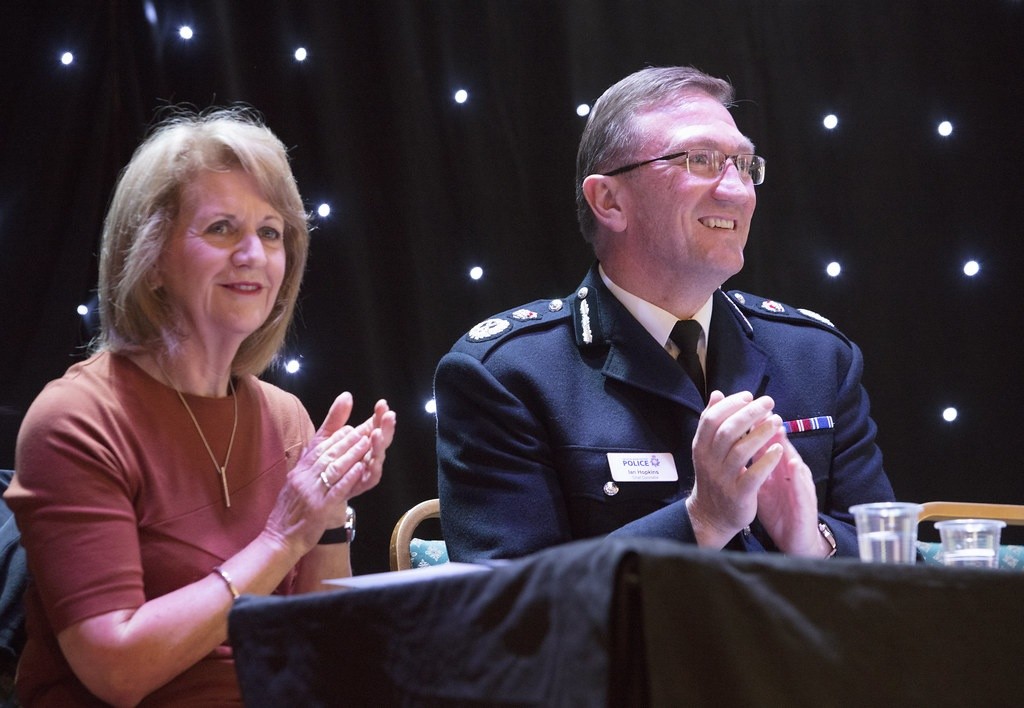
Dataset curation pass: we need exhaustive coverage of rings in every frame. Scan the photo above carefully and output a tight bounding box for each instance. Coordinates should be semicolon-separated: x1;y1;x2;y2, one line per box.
320;471;330;488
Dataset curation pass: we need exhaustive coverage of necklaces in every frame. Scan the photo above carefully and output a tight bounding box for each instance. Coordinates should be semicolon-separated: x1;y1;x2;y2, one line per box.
154;356;238;507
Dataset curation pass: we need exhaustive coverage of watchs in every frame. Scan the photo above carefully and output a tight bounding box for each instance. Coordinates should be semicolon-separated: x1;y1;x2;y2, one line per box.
317;506;356;544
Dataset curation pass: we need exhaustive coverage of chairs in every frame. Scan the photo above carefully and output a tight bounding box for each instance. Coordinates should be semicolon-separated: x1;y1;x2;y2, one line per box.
918;500;1024;528
390;498;450;573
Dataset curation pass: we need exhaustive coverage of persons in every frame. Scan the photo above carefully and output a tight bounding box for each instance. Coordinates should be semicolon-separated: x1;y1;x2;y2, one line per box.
3;109;398;708
435;67;897;564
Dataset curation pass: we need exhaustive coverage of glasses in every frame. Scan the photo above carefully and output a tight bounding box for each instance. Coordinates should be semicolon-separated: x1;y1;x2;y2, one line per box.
602;148;766;185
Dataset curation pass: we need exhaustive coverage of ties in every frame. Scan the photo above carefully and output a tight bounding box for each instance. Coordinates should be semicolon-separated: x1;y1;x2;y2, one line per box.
666;319;709;408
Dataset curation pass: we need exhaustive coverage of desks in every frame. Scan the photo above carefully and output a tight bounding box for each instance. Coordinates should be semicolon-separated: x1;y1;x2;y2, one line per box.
227;536;1024;708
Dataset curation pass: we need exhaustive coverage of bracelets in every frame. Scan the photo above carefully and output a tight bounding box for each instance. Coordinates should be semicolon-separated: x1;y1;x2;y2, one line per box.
818;523;836;559
212;567;239;598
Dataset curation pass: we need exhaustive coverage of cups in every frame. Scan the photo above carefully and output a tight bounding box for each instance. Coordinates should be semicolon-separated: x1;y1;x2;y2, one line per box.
933;518;1006;568
849;502;924;565
